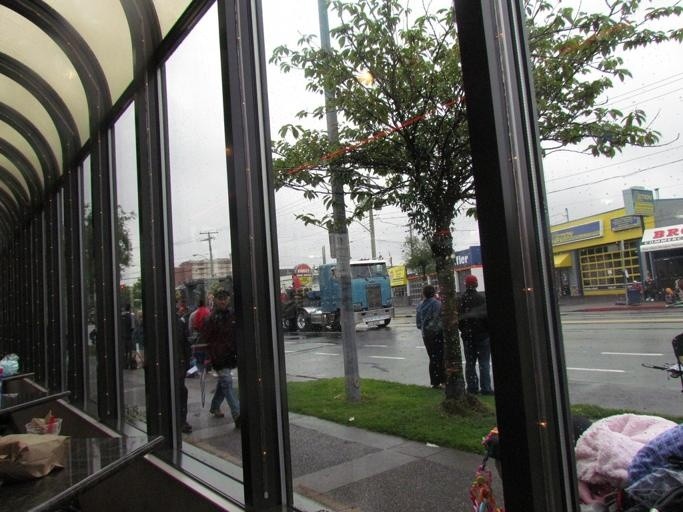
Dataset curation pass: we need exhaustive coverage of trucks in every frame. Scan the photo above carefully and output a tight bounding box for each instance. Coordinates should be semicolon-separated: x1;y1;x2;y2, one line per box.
281;259;397;334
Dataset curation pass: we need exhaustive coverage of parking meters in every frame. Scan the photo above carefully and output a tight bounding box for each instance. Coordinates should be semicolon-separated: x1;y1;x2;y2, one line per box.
622;270;630;305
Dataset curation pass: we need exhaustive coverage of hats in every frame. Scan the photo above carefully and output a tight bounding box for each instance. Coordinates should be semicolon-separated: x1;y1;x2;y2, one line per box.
463;274;478;284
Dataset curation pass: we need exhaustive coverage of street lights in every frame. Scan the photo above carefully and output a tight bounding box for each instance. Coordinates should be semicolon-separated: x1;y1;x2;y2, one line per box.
192;253;216;279
348;214;377;261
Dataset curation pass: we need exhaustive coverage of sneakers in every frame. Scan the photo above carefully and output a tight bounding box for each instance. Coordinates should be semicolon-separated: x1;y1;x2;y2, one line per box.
432;382;494;396
179;408;241;433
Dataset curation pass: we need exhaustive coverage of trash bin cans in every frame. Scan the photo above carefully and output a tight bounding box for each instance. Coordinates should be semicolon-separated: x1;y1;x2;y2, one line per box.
626;282;642;306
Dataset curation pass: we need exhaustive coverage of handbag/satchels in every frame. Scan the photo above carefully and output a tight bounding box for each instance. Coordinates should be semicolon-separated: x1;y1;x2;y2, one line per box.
129;351;144;371
422;318;445;347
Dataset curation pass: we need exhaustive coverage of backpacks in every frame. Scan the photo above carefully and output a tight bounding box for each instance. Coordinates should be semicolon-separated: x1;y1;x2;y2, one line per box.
191;309;208;329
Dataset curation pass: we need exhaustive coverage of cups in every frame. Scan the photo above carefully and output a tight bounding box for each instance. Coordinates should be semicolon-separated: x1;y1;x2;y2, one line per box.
25;418;62;435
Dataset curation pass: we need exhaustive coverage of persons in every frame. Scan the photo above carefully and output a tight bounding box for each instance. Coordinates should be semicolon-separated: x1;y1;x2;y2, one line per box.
413;284;446;389
88;289;241;436
456;275;496;395
